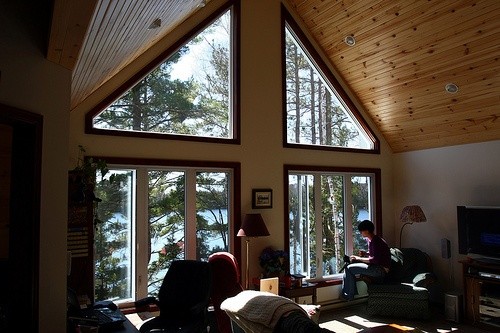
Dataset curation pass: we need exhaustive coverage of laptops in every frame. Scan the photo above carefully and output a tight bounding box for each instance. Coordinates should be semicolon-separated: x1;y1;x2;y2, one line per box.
67;289;126;325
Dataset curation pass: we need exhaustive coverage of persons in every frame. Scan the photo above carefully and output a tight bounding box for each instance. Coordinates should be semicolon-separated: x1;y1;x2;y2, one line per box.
341;220;391;301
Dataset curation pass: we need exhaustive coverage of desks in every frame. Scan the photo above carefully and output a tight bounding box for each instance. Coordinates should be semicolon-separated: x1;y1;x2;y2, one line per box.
280;283;317;306
94;301;141;333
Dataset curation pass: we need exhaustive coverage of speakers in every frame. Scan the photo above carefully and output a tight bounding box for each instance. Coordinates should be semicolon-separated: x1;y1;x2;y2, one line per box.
445;289;464;324
441;239;450;259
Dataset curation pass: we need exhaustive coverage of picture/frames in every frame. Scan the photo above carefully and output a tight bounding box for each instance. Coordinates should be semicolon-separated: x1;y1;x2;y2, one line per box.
252;188;273;210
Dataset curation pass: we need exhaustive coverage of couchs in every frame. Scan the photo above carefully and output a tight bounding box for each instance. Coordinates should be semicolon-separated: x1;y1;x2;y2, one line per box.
361;248;436;320
220;290;329;333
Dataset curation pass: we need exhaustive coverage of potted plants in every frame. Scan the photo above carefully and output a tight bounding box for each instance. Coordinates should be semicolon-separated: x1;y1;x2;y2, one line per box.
68;144;108;183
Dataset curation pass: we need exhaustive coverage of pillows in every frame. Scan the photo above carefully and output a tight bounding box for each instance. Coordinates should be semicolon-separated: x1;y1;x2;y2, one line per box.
298;303;322;326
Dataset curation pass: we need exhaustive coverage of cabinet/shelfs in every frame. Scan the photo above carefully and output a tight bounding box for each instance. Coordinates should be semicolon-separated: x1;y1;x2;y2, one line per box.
458;258;500;333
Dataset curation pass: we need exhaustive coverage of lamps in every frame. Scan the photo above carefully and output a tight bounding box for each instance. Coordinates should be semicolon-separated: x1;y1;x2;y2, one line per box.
236;213;270;289
399;205;428;248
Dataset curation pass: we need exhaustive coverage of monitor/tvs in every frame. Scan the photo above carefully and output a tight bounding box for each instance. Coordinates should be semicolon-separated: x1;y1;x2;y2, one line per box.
456;206;500;266
